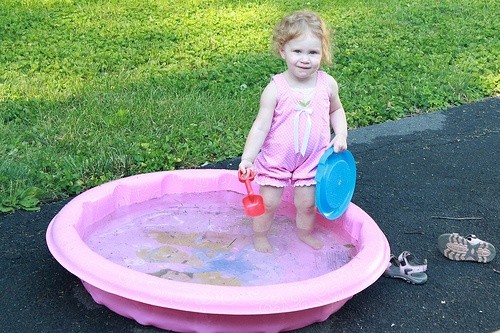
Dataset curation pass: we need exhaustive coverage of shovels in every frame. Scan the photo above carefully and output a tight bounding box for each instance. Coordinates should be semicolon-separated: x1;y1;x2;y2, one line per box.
238;169;265;218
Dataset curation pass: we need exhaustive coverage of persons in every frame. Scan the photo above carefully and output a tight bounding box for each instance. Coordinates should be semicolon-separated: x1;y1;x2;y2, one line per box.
238;10;348;253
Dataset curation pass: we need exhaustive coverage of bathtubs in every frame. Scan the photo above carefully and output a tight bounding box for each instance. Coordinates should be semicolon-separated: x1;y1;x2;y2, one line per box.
45;167;392;333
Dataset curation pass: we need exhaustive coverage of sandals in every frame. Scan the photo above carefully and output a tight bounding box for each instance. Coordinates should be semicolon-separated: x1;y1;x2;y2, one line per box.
438;232;496;264
383;250;429;285
